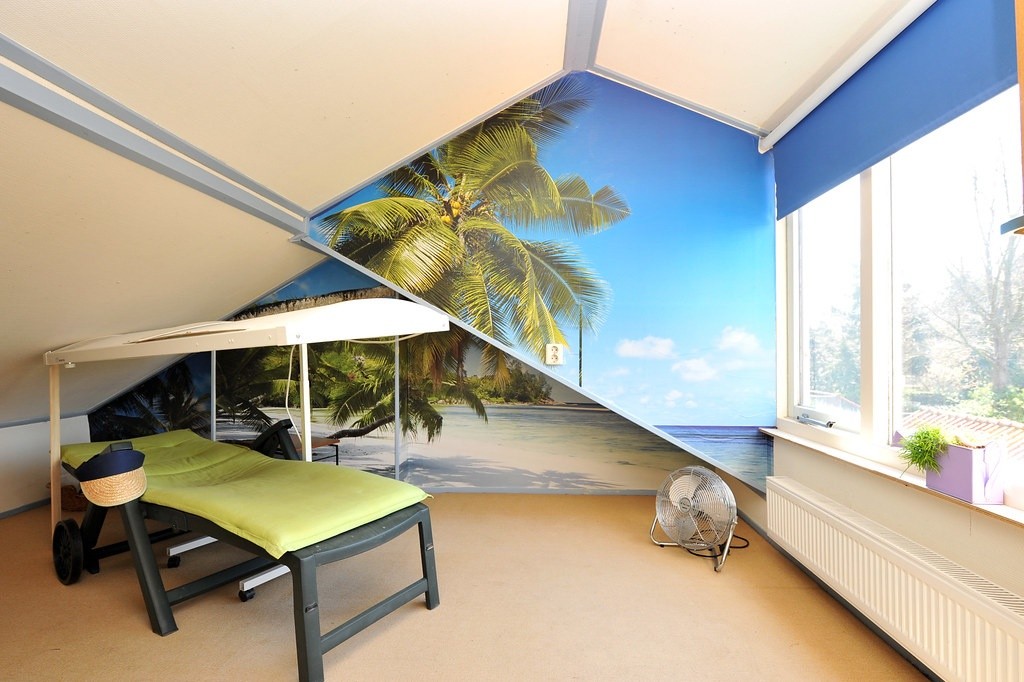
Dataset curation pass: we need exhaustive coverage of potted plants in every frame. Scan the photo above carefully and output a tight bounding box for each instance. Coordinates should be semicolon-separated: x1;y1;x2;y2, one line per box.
902;424;1004;506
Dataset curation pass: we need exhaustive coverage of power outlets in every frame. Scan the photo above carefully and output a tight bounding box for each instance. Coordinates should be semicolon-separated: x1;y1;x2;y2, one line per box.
545;345;563;365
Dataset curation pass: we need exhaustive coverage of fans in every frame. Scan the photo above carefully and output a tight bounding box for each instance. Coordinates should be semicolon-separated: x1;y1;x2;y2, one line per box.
649;465;736;572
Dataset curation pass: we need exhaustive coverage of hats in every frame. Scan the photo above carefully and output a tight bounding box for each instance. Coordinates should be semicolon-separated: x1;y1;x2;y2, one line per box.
74;449;147;507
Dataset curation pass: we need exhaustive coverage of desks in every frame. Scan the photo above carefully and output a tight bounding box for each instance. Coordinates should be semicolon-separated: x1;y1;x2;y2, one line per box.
275;433;341;464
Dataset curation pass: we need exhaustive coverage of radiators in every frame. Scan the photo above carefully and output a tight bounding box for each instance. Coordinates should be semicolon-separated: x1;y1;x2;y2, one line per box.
766;475;1024;682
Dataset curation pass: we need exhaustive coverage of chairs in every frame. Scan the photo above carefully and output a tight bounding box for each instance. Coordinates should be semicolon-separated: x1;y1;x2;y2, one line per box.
55;419;440;682
